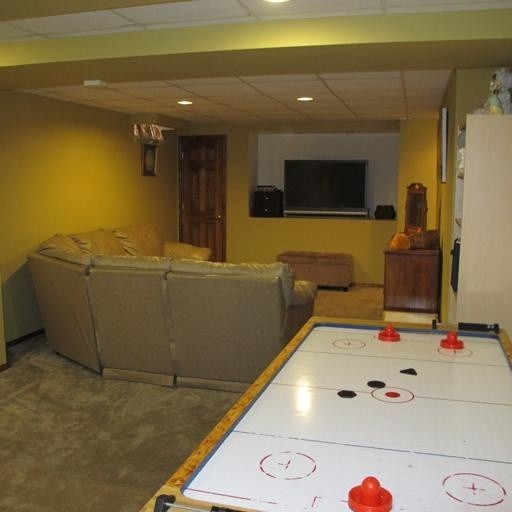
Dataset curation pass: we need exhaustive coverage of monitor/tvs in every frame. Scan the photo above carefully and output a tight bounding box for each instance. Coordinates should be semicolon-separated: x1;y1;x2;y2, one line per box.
283;160;368;216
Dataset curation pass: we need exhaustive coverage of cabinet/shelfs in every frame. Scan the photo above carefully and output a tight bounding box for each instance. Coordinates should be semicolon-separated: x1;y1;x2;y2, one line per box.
447;113;511;329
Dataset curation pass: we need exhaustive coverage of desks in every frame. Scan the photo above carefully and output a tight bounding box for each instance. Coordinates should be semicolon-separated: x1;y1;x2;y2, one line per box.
135;314;510;512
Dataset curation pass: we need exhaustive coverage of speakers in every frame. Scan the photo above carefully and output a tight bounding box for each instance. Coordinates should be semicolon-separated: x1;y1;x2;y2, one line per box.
254;192;282;217
374;206;396;219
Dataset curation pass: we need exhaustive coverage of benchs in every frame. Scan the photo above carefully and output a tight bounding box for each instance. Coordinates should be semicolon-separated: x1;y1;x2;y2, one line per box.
276;250;353;292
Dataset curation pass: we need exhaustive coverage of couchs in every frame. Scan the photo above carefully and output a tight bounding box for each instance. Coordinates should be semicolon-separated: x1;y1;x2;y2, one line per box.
26;226;320;393
